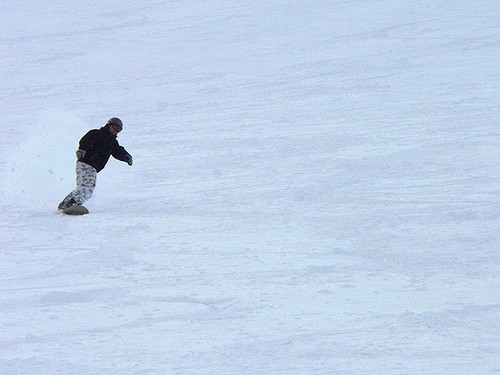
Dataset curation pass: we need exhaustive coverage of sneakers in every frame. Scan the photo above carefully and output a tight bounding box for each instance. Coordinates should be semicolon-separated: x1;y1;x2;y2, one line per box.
57;198;78;209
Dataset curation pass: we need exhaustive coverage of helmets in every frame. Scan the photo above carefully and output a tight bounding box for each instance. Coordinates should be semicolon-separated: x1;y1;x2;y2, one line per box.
107;117;122;132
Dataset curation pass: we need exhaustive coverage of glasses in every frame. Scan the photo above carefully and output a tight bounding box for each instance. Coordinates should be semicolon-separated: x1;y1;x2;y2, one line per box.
107;122;121;131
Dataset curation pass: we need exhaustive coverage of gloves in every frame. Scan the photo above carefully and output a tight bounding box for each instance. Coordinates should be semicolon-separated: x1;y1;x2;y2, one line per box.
124;154;133;166
76;150;86;160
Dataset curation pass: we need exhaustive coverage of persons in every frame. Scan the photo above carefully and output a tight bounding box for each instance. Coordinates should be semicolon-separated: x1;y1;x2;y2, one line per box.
57;117;132;209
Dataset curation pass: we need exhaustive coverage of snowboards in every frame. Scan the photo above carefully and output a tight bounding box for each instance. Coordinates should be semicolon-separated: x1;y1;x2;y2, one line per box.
57;206;89;215
